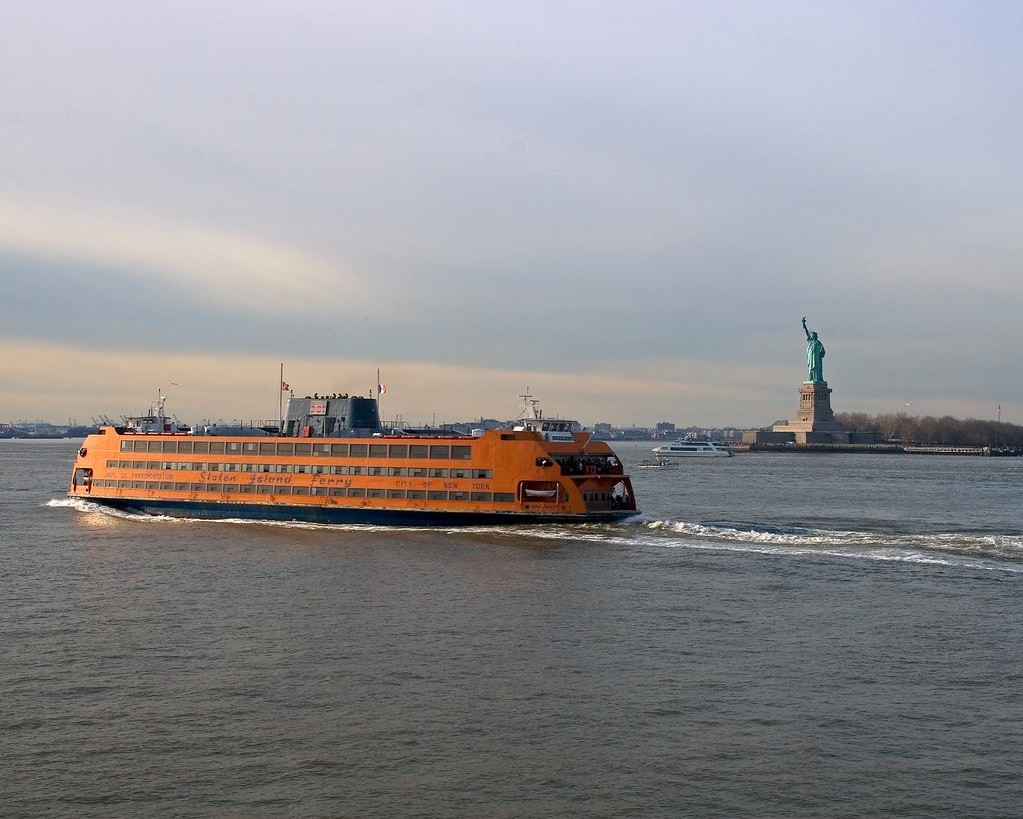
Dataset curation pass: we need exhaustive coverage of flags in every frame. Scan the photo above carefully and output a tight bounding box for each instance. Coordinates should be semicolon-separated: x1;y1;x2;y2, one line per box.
282;382;289;390
379;385;386;394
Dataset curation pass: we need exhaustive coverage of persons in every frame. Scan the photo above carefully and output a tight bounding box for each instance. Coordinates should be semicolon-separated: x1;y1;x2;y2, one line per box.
611;494;629;510
801;316;825;381
554;458;612;475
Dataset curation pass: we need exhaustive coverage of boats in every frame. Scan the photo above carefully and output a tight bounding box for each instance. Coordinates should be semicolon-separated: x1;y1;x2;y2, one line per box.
638;456;679;471
68;361;643;525
650;433;737;457
